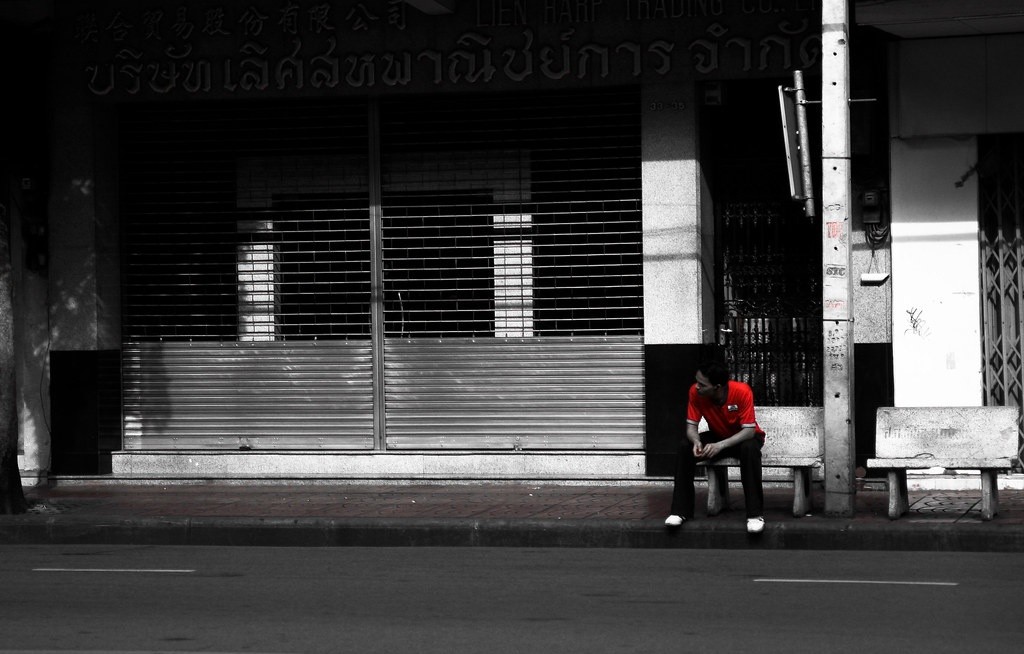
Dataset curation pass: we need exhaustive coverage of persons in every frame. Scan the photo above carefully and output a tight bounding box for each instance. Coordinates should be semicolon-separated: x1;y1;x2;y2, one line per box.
666;361;767;531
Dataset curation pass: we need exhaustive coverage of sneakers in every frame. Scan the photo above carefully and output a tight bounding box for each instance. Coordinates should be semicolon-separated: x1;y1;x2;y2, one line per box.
747;517;764;533
665;514;686;525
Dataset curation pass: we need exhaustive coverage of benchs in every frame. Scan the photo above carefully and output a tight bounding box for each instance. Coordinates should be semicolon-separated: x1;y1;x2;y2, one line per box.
867;405;1021;520
697;406;826;516
